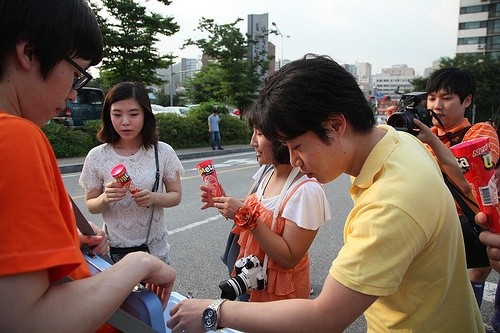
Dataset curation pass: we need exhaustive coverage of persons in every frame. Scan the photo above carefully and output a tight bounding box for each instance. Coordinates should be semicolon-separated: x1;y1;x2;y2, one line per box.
199;101;332;303
389;66;500;333
208;108;225;150
0;0;176;333
163;51;486;333
79;80;186;266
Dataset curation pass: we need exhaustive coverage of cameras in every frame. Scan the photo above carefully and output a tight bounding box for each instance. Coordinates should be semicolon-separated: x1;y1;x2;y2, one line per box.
387;91;433;135
218;253;269;301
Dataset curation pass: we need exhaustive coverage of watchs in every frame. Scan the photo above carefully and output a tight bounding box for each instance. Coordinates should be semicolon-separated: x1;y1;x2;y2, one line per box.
201;297;229;332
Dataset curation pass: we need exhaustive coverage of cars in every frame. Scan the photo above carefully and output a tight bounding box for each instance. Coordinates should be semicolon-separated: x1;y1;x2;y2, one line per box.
150;104;198;117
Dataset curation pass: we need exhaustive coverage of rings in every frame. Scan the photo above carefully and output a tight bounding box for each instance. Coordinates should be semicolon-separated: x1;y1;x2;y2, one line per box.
143;196;145;200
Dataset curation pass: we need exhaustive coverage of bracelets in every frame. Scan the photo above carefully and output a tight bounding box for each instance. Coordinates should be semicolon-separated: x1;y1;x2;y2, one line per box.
230;202;261;235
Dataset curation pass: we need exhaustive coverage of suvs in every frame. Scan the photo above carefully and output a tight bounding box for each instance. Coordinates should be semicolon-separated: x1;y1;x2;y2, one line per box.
55;87;106;127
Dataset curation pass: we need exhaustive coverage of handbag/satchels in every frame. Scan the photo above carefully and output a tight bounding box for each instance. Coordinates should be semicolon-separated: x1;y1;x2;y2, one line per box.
109;242;151;287
221;164;274;269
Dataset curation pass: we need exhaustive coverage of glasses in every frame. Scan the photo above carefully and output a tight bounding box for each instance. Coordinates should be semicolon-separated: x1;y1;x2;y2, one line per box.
42;40;93;90
